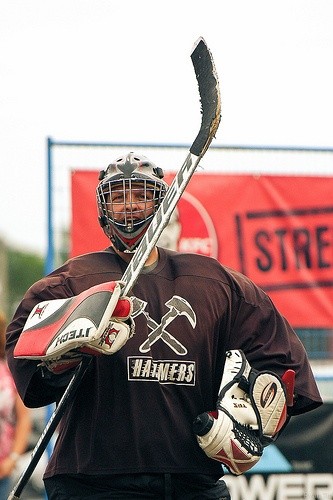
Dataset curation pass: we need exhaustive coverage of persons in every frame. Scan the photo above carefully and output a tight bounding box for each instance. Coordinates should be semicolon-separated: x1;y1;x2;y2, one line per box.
4;148;324;500
0;312;32;500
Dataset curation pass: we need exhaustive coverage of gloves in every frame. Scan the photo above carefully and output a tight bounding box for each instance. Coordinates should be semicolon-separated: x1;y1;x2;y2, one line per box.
14;281;131;374
195;348;293;476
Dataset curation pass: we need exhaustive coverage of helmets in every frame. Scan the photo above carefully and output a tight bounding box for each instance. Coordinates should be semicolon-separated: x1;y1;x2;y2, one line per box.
94;151;169;253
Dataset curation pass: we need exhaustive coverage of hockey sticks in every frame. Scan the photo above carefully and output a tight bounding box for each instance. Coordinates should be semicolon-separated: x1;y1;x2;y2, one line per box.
6;36;222;500
